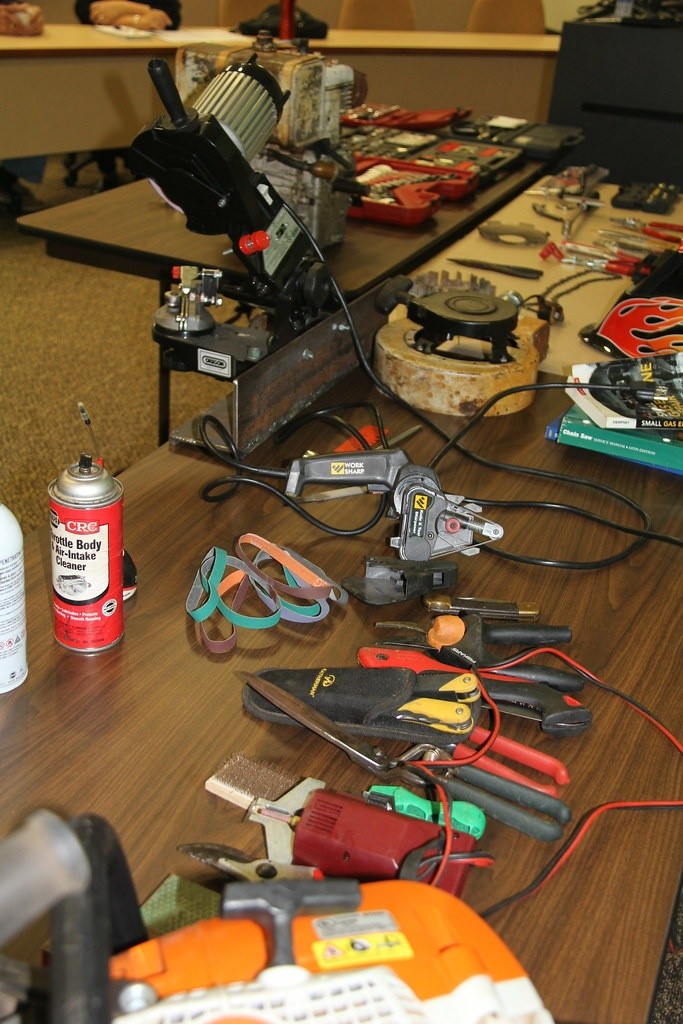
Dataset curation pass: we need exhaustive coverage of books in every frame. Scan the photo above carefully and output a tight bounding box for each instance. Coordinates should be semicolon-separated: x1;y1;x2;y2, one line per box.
544;352;683;482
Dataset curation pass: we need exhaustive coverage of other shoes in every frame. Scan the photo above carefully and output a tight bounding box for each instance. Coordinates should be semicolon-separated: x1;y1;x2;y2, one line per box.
0;185;47;212
101;174;119;191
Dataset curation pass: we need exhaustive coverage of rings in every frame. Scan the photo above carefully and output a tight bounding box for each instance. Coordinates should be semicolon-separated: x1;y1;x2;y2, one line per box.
12;24;15;29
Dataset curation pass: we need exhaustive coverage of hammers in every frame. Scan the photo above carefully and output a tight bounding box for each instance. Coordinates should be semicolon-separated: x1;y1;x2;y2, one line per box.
219;873;362;990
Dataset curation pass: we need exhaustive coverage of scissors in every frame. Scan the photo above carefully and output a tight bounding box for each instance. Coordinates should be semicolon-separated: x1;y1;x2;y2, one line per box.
237;668;570;843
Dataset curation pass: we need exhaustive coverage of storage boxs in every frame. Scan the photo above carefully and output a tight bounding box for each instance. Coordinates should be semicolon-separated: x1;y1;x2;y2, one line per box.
339;103;573;227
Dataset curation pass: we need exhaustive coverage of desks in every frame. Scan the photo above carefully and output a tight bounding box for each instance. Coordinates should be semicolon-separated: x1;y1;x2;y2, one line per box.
1;371;682;1024
388;176;683;379
0;27;562;160
15;125;554;447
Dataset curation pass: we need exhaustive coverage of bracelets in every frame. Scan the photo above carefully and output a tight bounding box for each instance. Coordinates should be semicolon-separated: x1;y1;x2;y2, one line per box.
133;14;140;28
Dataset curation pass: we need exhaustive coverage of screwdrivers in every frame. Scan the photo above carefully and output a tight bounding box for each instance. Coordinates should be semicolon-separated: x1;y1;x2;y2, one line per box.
370;423;424;451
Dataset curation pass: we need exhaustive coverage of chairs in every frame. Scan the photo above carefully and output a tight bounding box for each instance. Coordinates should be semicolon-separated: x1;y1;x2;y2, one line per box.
336;0;417;30
219;0;276;25
468;0;544;33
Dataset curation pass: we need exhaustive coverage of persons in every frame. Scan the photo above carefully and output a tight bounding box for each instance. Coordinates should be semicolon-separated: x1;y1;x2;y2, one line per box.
74;0;182;191
0;0;48;212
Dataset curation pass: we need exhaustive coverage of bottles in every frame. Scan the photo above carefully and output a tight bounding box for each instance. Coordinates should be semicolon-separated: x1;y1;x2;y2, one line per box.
0;503;28;696
46;454;126;653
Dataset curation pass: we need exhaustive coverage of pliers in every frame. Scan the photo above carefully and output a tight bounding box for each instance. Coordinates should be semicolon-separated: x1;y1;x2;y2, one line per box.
176;840;326;888
608;216;683;244
560;256;651;276
446;257;543;279
436;724;571;797
373;613;586;694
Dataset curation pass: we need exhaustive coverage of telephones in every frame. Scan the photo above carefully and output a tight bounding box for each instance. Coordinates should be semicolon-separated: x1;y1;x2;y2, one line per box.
240;2;328;39
